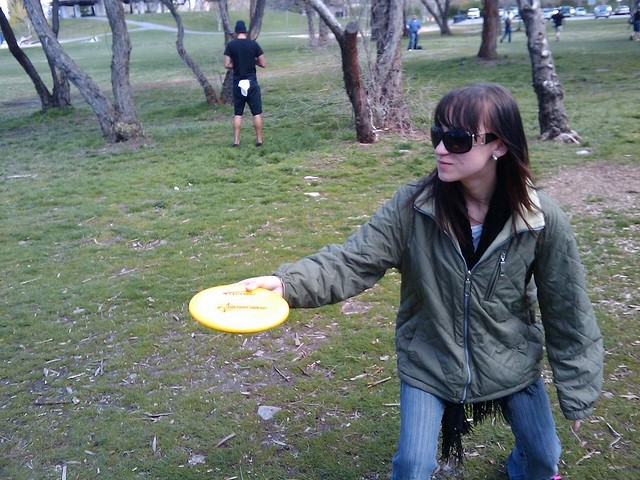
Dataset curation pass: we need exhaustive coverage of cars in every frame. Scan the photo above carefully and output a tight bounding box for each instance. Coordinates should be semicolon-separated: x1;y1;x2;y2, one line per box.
615;6;630;15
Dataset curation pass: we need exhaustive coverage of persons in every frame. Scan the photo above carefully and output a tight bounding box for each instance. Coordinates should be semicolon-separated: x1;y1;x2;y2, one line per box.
239;85;604;479
222;21;267;148
405;14;423;52
499;17;512;44
548;9;564;40
628;7;640;40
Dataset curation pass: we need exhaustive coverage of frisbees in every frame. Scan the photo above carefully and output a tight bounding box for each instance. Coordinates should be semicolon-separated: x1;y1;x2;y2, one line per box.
188;283;289;334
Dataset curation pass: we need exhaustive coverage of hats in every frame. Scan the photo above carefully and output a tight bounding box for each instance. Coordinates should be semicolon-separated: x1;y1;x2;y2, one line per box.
235;20;246;33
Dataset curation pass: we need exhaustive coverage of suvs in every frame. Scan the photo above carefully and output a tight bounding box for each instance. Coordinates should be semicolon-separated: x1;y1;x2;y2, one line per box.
468;8;481;19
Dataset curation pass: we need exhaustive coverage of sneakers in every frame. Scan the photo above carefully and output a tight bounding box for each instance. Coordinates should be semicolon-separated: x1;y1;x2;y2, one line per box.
408;48;412;51
256;141;263;147
233;143;239;147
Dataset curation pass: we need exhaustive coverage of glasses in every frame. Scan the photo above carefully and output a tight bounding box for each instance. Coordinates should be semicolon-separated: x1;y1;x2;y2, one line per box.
430;126;498;154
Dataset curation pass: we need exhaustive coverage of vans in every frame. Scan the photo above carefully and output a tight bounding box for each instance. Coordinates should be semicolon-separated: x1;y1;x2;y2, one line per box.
594;5;612;18
576;7;586;16
